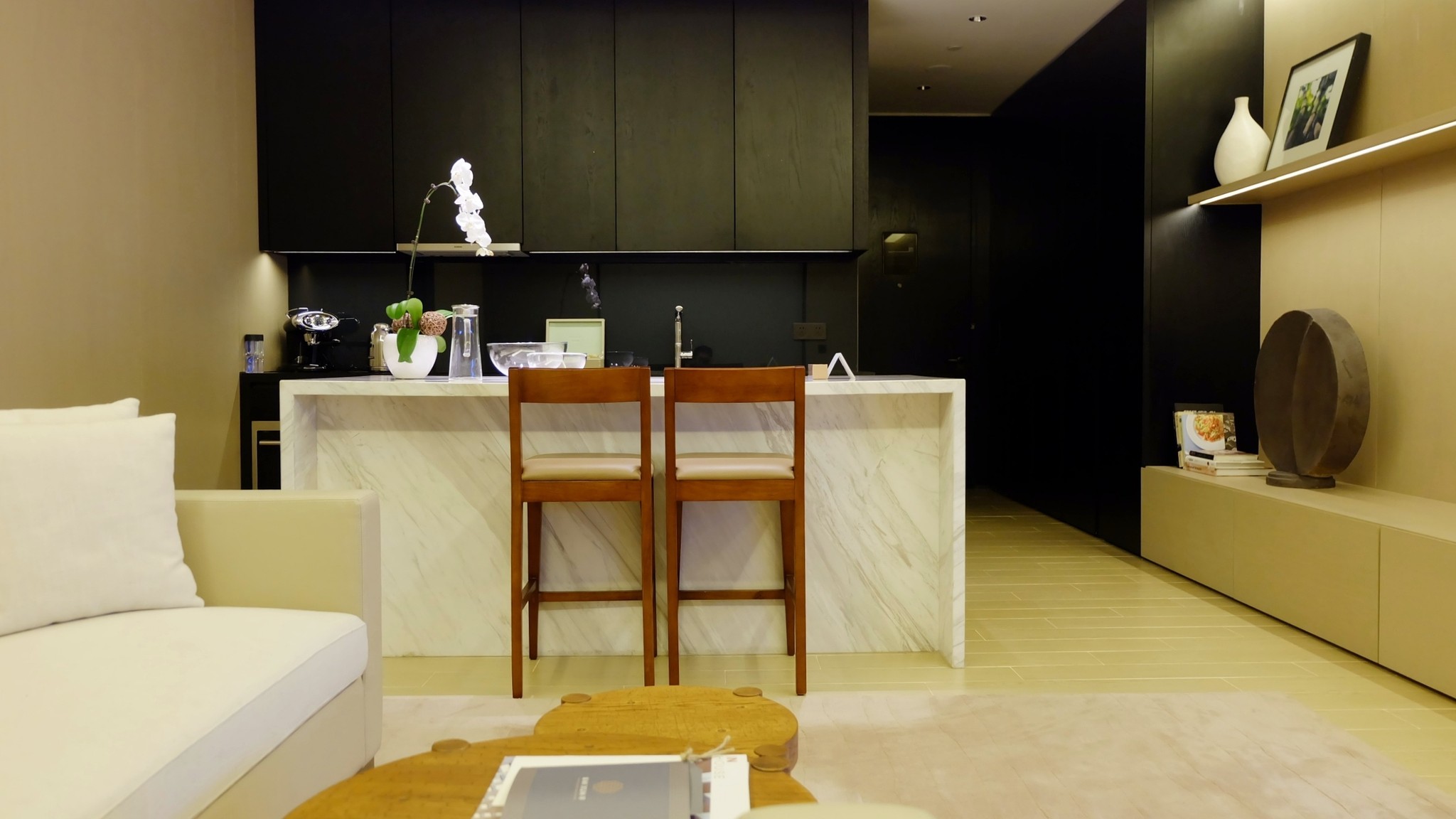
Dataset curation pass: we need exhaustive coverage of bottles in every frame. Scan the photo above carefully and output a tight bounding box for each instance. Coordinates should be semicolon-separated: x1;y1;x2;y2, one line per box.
368;322;390;372
1213;95;1271;186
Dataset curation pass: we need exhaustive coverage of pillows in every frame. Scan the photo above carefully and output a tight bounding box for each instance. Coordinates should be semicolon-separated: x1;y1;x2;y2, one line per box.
0;412;207;636
0;394;138;430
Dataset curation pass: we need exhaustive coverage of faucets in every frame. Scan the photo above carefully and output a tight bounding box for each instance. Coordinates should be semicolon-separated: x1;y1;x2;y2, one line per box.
674;305;695;368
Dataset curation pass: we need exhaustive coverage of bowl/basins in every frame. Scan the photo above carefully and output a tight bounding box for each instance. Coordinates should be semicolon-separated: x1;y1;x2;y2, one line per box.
526;352;587;369
487;342;568;376
603;351;649;367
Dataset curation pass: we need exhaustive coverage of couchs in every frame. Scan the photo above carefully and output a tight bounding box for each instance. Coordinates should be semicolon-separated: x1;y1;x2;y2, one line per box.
0;489;384;818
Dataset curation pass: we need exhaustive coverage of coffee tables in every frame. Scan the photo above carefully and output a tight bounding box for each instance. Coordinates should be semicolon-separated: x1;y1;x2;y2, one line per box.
287;683;821;819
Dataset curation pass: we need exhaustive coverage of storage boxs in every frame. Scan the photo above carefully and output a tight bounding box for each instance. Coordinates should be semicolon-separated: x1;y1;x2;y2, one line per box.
546;318;605;368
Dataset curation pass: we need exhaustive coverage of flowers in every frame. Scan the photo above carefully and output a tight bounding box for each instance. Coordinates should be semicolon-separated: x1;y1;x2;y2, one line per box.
386;157;494;362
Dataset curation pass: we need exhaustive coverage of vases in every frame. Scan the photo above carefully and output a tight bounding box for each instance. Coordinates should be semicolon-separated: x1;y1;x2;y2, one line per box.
1214;97;1270;187
380;333;438;379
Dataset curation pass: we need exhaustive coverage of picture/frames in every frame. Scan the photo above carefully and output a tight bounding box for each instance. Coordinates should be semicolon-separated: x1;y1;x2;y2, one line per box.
1264;32;1371;172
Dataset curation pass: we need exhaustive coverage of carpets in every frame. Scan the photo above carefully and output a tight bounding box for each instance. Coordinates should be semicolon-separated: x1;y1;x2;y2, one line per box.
377;696;1456;819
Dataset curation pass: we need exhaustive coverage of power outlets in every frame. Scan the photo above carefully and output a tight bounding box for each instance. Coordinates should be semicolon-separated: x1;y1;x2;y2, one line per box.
794;322;828;341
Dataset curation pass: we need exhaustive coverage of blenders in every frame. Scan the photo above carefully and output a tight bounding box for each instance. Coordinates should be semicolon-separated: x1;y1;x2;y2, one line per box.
283;306;340;371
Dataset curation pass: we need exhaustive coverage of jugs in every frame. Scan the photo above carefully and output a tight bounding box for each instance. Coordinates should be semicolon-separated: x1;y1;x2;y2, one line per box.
448;305;483;383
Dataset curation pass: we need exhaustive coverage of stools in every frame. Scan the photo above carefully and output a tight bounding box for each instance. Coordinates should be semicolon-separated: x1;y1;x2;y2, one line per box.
662;365;806;697
508;364;655;699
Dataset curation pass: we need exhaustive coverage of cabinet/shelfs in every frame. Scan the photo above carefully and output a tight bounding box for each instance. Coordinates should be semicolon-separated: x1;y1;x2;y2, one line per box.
253;0;871;257
1138;108;1456;696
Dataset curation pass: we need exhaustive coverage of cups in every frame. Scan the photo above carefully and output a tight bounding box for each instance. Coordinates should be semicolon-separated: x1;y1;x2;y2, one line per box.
243;334;265;374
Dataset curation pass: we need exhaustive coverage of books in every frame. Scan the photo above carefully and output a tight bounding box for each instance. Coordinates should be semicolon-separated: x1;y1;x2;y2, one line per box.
1174;410;1276;476
